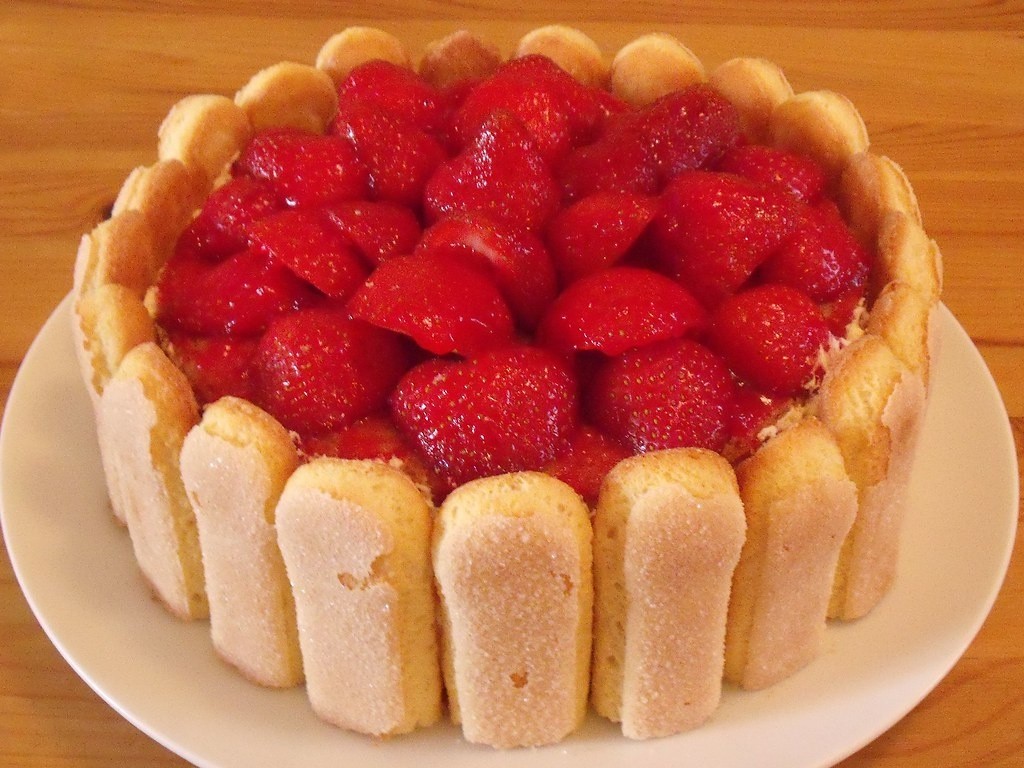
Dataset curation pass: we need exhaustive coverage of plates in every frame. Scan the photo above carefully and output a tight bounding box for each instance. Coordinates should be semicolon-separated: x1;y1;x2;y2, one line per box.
0;247;1021;768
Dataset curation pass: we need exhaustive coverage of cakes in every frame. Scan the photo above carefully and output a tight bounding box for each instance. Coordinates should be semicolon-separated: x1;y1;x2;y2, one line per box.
76;22;946;747
